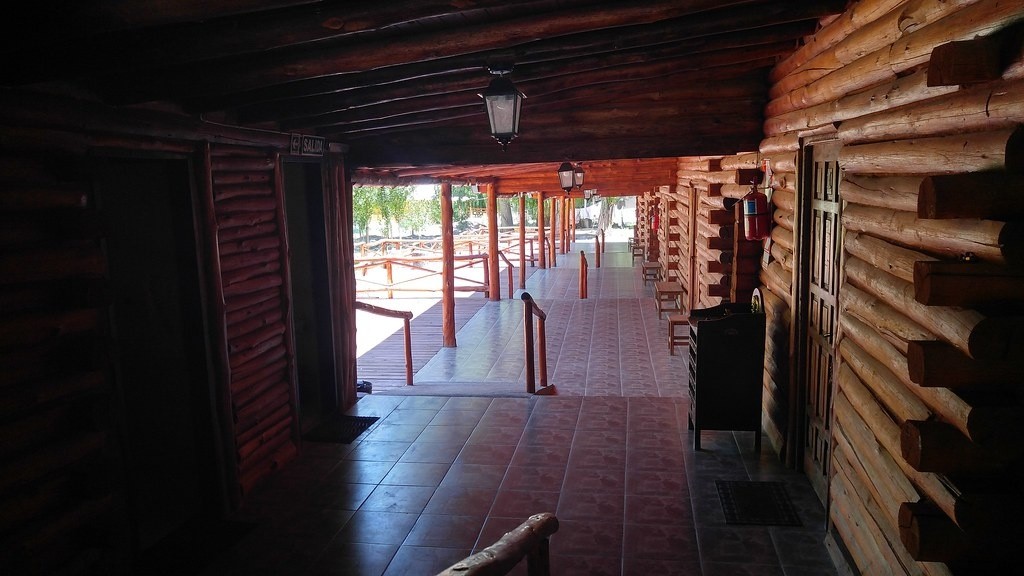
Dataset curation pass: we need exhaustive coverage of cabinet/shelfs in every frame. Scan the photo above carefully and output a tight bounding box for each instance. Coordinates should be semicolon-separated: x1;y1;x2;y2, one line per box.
688;287;767;455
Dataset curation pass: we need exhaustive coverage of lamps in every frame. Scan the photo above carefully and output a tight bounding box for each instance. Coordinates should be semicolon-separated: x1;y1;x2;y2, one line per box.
557;162;602;204
478;61;528;153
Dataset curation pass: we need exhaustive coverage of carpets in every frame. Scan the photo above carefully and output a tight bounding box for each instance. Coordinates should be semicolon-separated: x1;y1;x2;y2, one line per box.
714;479;803;527
302;413;380;444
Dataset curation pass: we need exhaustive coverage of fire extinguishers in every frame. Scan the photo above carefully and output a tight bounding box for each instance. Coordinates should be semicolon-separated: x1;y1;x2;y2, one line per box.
650;210;658;229
742;176;772;241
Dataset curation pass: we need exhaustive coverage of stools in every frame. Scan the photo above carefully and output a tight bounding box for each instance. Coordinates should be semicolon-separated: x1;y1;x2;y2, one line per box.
628;237;646;268
641;261;662;287
654;280;684;321
667;314;691;356
